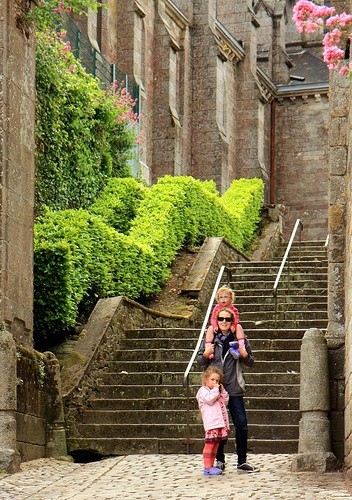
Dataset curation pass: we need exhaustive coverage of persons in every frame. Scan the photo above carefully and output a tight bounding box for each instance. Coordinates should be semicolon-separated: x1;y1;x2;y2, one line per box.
197;308;260;472
195;364;230;475
203;287;248;360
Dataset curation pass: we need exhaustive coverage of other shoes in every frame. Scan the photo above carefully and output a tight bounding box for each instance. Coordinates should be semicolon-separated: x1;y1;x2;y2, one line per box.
213;461;225;470
237;463;260;473
204;467;222;475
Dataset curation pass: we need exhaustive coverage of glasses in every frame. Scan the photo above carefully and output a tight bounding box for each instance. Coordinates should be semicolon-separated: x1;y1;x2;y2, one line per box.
217;316;232;322
218;296;229;300
